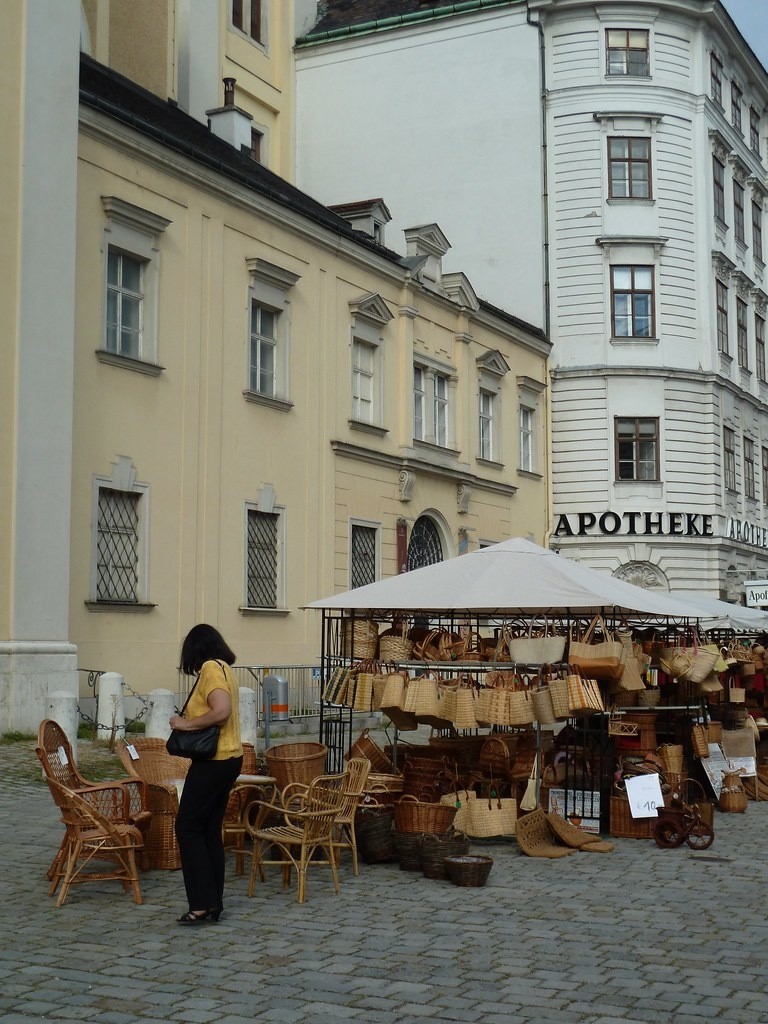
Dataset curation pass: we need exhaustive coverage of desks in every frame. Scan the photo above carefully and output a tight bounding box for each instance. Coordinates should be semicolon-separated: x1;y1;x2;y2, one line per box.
222;774;277;882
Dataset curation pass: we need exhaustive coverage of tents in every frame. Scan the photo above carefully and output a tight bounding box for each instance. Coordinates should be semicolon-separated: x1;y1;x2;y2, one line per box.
297;538;768;667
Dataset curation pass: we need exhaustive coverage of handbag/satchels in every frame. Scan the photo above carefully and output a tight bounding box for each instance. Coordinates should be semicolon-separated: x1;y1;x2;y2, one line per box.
165;659;227;761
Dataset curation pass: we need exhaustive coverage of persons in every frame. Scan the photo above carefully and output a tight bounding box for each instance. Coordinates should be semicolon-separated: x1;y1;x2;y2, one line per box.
169;624;243;925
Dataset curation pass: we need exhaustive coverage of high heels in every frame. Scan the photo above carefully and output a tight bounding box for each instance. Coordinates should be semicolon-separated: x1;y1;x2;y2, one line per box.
175;907;224;924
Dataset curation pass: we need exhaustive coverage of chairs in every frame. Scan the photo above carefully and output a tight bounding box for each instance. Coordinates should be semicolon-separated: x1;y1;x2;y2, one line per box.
281;758;372;882
115;737;192;870
43;776;144;908
38;718;153;879
243;771;351;904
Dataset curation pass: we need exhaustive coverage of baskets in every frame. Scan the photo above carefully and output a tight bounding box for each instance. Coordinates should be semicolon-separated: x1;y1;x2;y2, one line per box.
718;767;748;813
264;608;768;842
